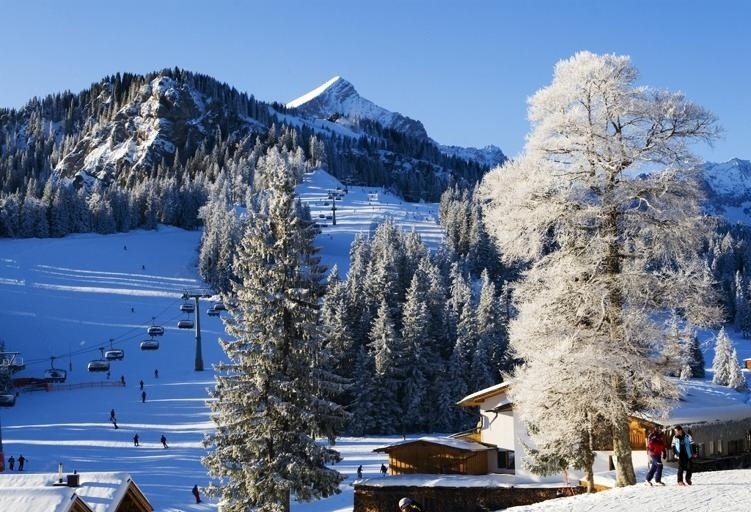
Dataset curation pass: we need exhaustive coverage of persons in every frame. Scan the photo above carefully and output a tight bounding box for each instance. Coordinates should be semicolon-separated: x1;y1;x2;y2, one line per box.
140;380;145;389
192;484;201;503
18;454;25;470
111;416;119;429
109;409;116;420
357;464;364;479
8;455;16;469
399;497;422;511
132;433;140;446
154;368;159;377
380;463;389;476
646;423;667;486
121;375;124;382
673;424;698;486
106;371;111;379
160;434;168;447
141;390;146;404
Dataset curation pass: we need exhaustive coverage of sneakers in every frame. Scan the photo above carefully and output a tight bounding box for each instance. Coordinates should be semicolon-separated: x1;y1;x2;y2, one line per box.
655;482;665;487
678;481;685;486
645;480;652;487
686;480;692;485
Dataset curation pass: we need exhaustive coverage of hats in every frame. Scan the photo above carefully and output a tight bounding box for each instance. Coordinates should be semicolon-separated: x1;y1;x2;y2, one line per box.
399;497;411;510
